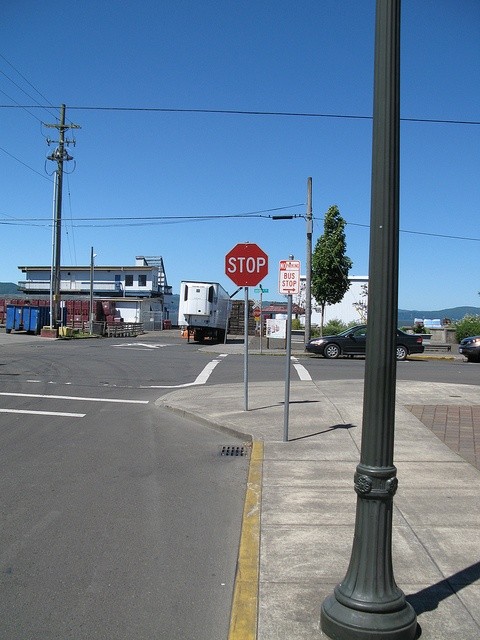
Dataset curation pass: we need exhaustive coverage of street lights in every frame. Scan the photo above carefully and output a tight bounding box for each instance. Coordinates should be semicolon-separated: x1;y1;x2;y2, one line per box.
92;254;98;320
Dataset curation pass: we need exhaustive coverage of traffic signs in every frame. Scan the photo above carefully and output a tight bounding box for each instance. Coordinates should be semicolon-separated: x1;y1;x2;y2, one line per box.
279;260;301;296
254;289;269;293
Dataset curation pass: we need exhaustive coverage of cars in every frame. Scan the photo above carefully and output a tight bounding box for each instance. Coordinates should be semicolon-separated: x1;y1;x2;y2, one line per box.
305;325;424;361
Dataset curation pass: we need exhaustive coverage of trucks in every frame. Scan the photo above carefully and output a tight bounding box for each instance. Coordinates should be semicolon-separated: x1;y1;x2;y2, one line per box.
178;281;234;344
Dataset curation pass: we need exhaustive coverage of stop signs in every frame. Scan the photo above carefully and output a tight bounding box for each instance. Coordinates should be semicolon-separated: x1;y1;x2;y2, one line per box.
253;309;261;317
225;243;269;287
